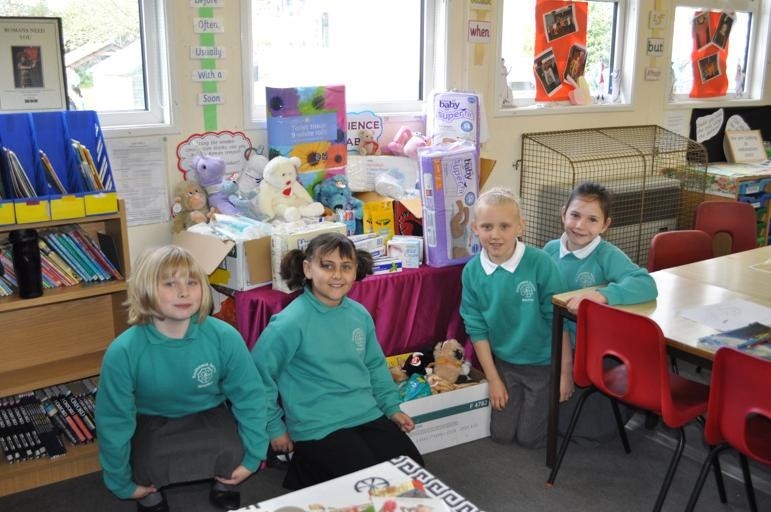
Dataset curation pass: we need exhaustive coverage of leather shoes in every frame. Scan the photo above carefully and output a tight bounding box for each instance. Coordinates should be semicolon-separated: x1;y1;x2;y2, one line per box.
210;489;240;510
138;494;169;512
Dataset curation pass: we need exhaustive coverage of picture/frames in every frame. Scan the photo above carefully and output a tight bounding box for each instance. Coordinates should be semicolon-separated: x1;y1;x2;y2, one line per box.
0;16;68;114
725;129;767;164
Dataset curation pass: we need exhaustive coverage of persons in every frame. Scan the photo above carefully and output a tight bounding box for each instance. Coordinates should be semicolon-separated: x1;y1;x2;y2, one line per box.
542;180;659;444
538;13;586;91
458;187;576;447
695;15;728;78
94;243;270;510
17;54;36;88
249;232;424;489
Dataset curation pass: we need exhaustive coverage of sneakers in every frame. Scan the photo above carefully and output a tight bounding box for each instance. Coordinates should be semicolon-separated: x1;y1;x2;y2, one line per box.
644;411;660;429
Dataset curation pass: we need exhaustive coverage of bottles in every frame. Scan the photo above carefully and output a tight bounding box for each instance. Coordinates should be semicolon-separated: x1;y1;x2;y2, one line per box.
570;51;584;79
10;230;44;299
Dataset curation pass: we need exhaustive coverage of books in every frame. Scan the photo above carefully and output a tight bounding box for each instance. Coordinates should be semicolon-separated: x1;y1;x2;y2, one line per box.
0;375;101;464
699;321;770;359
0;138;123;297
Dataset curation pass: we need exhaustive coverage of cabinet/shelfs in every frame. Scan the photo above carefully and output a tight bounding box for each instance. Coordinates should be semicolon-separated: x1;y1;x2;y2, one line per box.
0;199;131;499
681;164;771;248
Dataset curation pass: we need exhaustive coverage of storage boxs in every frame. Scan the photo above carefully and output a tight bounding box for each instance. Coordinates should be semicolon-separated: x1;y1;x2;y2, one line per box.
169;212;274;292
395;382;492;457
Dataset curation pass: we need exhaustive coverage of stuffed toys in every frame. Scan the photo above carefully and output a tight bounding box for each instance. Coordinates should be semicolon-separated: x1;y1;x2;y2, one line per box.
358;129;381;156
381;127;432;157
389;339;479;401
171;149;364;234
